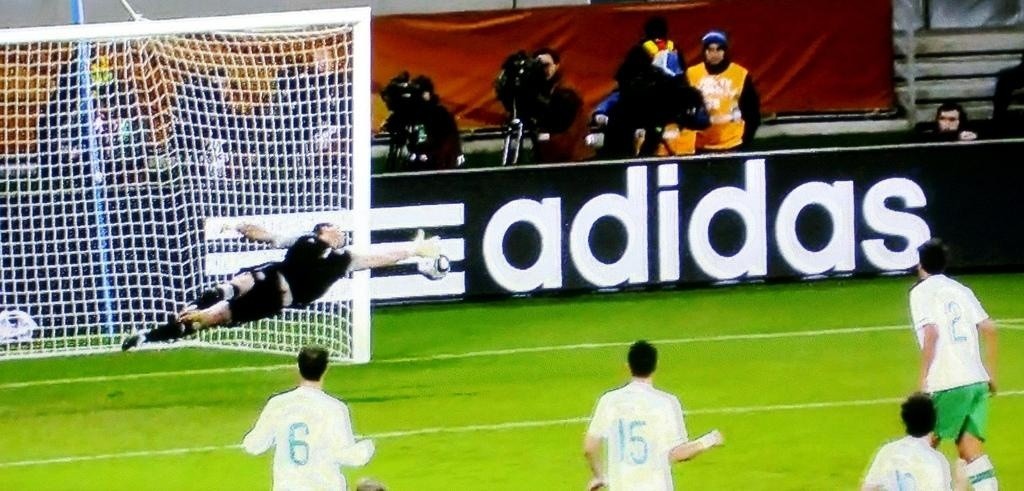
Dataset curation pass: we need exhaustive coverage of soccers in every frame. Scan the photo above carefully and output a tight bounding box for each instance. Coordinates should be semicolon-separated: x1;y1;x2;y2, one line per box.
418;255;451;281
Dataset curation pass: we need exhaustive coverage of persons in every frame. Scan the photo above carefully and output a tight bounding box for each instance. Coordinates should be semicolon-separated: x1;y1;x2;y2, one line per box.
584;338;725;491
907;239;1004;490
523;45;594;167
383;75;462;174
591;48;712;158
860;392;953;491
122;219;443;351
682;25;760;156
243;347;378;491
915;102;979;143
613;15;686;90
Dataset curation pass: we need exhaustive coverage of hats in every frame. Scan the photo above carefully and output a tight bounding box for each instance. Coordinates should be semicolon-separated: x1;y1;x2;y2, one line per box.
700;30;726;44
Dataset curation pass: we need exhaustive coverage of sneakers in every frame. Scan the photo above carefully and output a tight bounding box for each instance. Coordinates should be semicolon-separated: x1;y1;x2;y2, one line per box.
122;328;152;351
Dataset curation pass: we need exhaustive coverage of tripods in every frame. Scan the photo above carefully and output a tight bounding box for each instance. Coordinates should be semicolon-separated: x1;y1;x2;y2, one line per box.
501;98;542;168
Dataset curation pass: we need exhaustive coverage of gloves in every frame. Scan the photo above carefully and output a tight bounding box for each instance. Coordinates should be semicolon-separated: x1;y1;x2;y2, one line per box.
412;228;442;259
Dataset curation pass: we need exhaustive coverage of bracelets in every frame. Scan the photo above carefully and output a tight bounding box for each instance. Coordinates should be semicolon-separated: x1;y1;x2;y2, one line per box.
697;434;715;450
